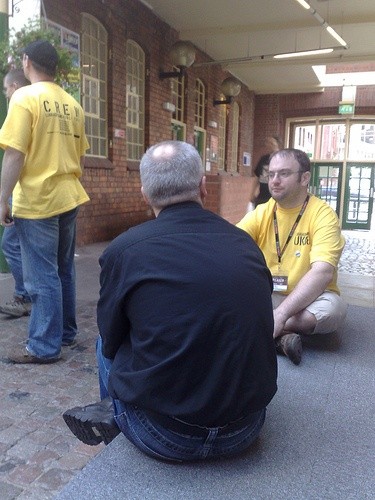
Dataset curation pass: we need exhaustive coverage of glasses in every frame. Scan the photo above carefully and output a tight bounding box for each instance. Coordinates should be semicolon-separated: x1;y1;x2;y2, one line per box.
268;169;306;179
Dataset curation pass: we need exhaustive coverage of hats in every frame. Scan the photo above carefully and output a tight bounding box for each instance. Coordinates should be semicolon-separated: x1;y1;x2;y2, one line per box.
14;39;59;75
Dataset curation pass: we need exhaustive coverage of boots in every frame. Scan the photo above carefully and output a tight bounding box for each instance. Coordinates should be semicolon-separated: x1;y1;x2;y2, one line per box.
63;396;120;446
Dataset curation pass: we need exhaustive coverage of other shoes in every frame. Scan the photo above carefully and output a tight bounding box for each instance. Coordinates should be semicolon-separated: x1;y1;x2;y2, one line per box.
274;332;301;365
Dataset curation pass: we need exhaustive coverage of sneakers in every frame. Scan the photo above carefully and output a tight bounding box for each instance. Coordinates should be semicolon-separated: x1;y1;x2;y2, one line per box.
7;347;60;365
0;296;32;317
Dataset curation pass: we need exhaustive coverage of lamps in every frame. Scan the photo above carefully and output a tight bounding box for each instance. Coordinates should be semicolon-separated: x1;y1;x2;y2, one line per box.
193;0;350;69
214;77;241;107
159;40;196;81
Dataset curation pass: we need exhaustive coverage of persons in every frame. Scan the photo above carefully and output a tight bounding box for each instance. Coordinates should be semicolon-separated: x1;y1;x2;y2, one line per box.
235;148;346;366
0;68;33;316
6;40;89;364
249;136;280;210
63;140;278;465
